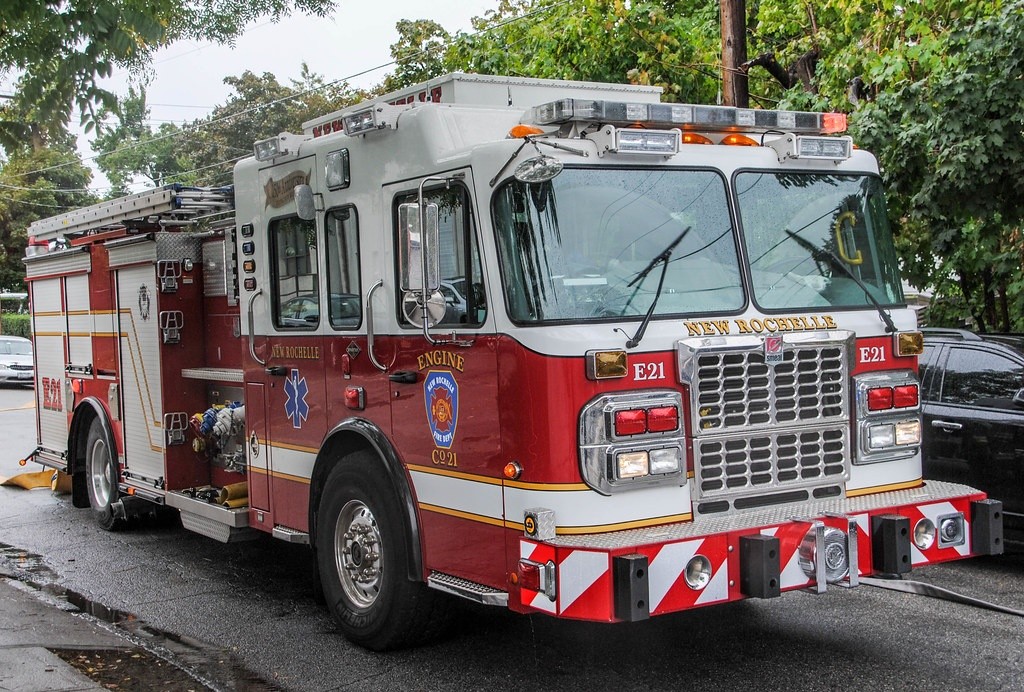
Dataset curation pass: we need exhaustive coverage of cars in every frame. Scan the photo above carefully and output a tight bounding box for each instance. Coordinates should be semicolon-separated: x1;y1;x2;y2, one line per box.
0;335;34;388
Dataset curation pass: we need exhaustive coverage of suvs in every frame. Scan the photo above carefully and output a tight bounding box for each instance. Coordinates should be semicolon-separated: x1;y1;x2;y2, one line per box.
917;326;1024;548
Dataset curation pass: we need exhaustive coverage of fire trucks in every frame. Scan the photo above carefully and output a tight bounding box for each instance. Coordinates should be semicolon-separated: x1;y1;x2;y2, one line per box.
20;69;1006;655
